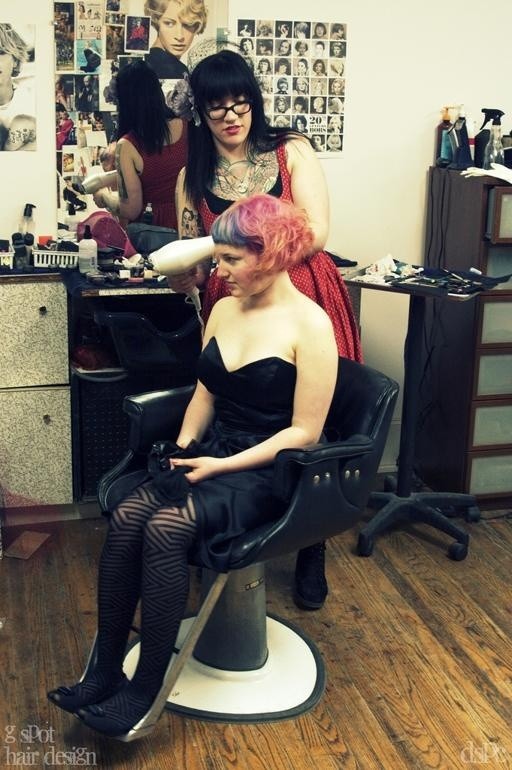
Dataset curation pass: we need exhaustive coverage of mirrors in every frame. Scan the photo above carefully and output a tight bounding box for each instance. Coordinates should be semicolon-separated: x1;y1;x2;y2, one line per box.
53;1;230;246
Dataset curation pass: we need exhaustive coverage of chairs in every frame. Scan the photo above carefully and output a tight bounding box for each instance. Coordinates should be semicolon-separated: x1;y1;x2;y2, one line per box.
70;355;400;744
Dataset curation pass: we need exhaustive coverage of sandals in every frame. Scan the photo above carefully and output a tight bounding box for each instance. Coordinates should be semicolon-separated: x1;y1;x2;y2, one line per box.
47;674;131;737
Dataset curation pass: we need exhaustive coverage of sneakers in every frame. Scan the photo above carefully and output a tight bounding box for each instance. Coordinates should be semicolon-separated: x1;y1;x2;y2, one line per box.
295;542;328;610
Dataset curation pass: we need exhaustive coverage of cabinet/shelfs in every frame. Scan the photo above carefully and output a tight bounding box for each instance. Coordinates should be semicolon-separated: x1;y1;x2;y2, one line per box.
0;271;74;516
400;165;512;515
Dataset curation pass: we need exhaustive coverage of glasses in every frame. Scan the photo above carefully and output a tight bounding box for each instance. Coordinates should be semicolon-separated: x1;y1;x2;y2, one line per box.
202;101;252;120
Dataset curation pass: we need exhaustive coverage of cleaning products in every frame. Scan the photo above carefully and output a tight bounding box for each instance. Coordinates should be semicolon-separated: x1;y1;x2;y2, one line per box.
19;203;37;234
479;107;506;168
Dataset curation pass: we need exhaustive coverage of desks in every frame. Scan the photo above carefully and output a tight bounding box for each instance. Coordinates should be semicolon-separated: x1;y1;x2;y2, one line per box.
74;260;361;331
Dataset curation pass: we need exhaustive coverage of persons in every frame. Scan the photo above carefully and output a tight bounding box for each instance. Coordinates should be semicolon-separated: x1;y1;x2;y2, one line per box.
43;189;342;735
0;21;36;153
53;0;212;233
166;47;365;611
238;18;346;153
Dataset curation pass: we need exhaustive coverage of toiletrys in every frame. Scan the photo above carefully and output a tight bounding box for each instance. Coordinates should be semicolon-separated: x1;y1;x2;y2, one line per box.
437;102;473;165
79;224;97;273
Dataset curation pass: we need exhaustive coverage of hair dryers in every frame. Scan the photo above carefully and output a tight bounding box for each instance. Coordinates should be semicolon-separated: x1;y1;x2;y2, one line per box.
148;235;214;311
81;170;120;212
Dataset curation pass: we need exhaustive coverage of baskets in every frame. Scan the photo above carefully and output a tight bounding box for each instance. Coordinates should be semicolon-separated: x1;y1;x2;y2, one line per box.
31;250;78;269
0;246;16;270
